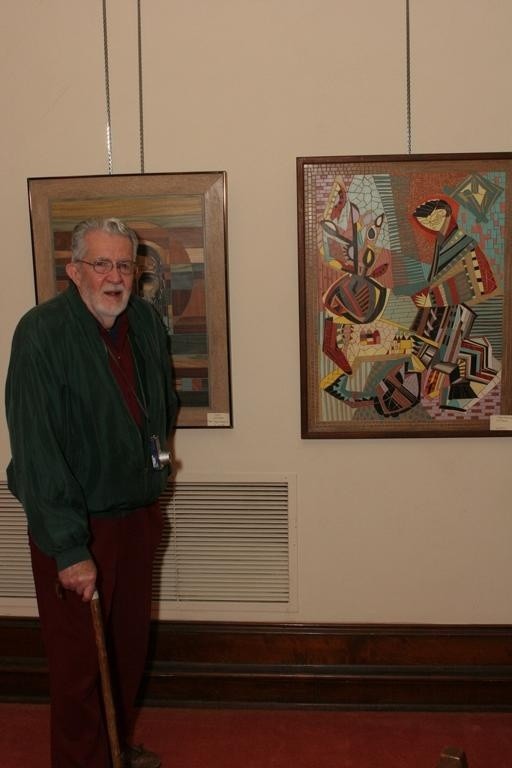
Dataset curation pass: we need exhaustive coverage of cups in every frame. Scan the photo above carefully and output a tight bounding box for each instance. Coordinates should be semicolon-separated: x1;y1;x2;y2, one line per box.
75;258;141;277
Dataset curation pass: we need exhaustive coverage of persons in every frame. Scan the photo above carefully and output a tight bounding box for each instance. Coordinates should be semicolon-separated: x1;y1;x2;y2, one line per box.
2;213;183;767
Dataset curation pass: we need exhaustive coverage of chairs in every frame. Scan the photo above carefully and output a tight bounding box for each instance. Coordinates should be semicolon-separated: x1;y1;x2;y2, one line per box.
109;740;162;767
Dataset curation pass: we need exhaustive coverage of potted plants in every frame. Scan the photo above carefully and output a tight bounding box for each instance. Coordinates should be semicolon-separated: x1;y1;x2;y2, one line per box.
28;171;232;429
297;152;512;439
